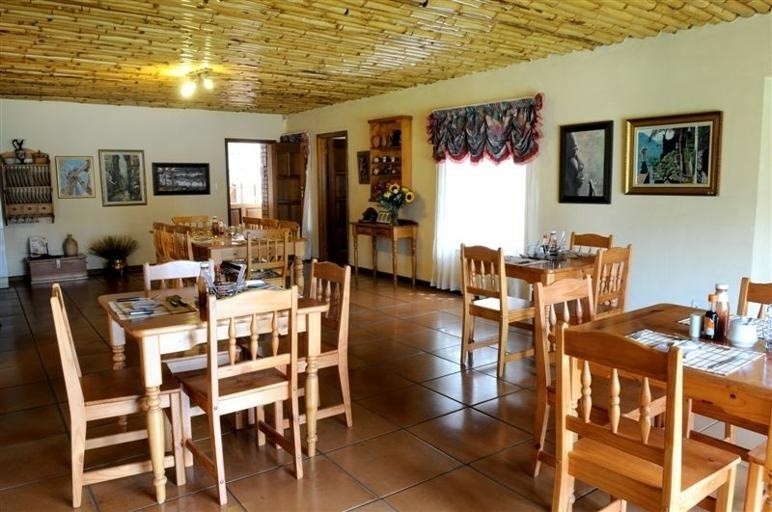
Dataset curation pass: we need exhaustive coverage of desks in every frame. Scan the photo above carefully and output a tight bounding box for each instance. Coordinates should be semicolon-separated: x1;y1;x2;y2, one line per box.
97;273;329;506
507;252;593;355
559;302;771;431
351;218;419;291
171;224;310;295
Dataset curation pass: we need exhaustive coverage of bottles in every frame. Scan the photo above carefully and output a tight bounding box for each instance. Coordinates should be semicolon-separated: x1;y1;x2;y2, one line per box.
213;264;223;285
207;216;224;238
198;264;212;307
764;303;771;349
542;232;557;261
688;282;730;340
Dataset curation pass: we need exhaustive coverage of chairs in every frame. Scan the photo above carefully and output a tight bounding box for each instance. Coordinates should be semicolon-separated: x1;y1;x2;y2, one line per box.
238;216;301;238
174;284;306;507
244;231;292;279
172;215;210;229
741;419;772;512
723;277;772;442
592;247;634;317
527;283;669;503
457;241;535;377
564;230;616;251
43;280;187;506
153;220;193;262
257;259;354;449
140;260;214;292
549;322;743;512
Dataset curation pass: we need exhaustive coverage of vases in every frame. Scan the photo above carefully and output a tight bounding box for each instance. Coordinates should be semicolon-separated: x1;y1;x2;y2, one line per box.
387;210;401;225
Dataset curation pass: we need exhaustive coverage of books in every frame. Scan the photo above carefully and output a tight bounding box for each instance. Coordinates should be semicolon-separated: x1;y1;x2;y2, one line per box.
27;236;48;258
108;294;196;320
197;261;284;299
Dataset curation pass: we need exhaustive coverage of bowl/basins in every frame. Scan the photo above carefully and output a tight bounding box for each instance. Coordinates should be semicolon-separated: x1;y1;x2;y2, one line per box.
4;158;16;164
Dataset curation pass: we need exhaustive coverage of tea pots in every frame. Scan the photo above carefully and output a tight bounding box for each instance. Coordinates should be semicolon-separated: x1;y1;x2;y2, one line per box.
11;138;27;164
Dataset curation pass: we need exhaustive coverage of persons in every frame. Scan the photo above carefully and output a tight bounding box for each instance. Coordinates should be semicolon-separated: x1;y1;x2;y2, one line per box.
158;167;206;190
566;132;597;197
65;160;92;197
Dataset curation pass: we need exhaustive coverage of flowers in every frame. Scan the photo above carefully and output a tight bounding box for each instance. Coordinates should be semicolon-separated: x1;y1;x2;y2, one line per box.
374;179;411;208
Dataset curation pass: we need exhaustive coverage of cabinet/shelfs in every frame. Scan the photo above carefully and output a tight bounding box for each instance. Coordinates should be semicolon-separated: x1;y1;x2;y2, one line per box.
367;116;411;201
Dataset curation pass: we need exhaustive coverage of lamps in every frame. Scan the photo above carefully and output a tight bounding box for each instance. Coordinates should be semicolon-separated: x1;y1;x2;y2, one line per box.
180;67;213;97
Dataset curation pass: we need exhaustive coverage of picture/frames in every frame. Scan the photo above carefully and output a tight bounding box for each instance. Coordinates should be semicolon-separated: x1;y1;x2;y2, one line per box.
151;161;211;196
622;111;722;197
553;119;613;205
53;154;97;201
98;150;147;206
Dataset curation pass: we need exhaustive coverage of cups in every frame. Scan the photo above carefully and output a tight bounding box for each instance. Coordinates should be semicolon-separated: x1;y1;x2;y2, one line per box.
527;240;536;256
727;319;758;349
372;167;399;175
373;155;401;163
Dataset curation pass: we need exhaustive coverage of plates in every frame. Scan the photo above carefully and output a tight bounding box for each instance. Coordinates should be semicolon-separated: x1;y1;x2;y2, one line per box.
371;130;399;148
17;159;34;164
